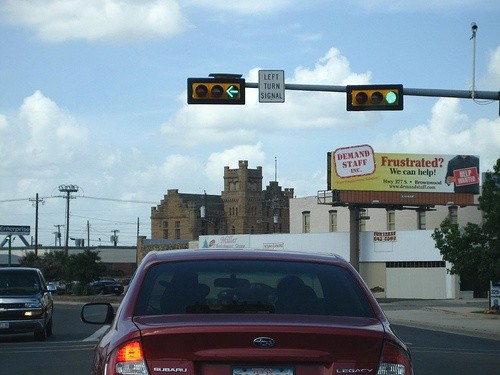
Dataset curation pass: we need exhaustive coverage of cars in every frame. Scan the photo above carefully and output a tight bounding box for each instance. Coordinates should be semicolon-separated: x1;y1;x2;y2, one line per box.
80;248;413;375
91;280;125;295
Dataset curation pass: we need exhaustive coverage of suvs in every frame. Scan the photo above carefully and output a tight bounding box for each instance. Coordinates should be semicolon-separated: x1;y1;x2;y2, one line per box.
0;268;57;340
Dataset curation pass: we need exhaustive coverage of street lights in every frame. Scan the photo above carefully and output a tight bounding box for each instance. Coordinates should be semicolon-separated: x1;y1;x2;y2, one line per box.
59;184;78;260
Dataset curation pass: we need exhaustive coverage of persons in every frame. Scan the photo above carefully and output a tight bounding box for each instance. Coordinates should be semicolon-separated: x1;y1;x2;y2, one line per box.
445;155;480;193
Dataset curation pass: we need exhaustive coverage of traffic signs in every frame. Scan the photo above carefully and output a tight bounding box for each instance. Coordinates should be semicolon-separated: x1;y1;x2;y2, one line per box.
257;69;284;101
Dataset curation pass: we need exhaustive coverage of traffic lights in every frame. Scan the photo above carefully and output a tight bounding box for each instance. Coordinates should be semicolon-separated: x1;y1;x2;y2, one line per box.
346;86;403;109
188;78;246;105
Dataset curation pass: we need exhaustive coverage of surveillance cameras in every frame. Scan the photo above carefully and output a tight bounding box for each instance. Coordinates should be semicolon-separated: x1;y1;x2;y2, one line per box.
470;22;479;31
